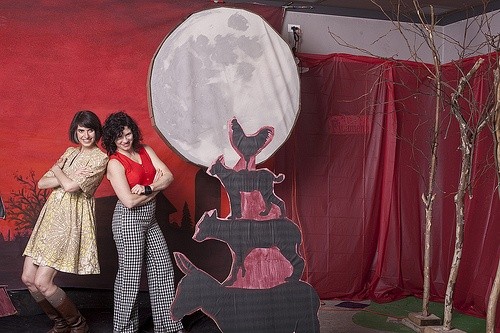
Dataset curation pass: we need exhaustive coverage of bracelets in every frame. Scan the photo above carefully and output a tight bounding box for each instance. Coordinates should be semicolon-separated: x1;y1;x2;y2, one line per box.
144;185;153;196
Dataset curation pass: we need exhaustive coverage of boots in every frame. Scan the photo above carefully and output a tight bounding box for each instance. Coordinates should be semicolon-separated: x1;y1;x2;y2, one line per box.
30;289;71;332
46;285;90;332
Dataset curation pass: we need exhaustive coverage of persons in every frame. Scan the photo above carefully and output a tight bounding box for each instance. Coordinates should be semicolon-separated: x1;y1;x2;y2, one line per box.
100;111;184;333
20;111;109;333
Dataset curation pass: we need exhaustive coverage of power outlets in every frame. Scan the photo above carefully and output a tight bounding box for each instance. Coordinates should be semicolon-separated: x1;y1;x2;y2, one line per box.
287;23;300;32
